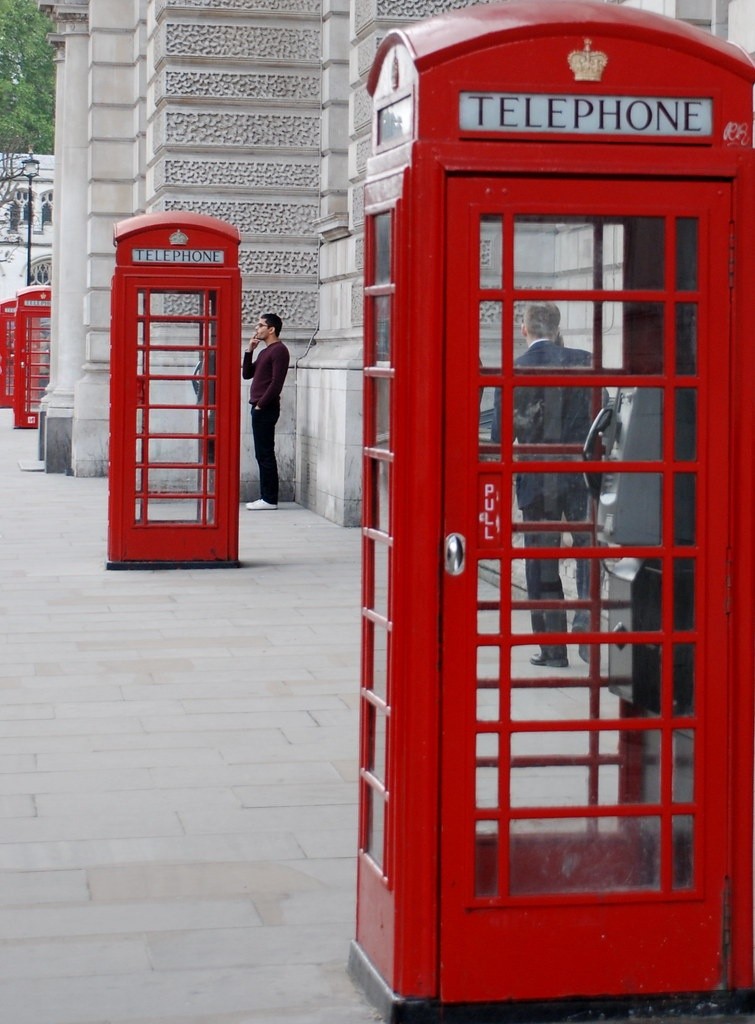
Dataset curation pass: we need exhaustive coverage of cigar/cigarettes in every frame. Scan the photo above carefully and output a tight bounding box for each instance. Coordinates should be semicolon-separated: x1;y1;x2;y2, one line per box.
251;331;257;334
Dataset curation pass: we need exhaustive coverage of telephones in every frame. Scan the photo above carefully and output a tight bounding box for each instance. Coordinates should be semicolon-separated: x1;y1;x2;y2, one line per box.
192;353;215;406
582;385;666;548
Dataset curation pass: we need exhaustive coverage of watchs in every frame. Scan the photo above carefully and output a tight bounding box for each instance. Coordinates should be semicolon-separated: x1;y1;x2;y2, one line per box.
243;348;250;353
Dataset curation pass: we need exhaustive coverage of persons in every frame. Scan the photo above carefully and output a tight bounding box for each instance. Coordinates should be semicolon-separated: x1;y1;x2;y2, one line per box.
241;313;290;510
489;301;609;668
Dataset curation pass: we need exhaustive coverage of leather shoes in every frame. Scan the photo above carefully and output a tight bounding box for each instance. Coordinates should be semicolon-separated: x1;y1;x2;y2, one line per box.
570;612;590;661
530;653;568;666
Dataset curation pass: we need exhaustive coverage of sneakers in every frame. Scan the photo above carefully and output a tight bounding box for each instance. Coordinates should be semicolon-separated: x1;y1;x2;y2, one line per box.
246;499;278;509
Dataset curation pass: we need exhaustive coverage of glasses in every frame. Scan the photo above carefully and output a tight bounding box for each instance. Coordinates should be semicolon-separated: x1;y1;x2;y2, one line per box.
257;323;268;327
